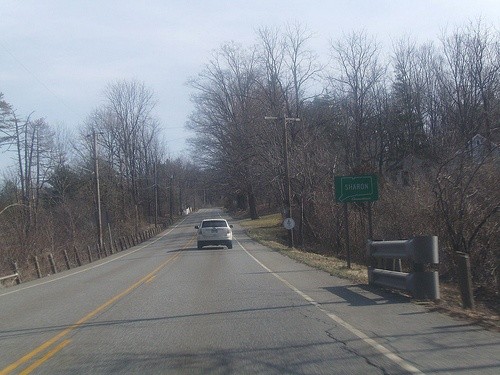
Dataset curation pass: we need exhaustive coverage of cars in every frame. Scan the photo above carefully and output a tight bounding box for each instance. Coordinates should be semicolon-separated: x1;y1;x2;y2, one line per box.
195;219;233;249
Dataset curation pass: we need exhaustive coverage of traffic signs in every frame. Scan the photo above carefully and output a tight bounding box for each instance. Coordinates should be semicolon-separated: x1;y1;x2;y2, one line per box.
334;175;379;202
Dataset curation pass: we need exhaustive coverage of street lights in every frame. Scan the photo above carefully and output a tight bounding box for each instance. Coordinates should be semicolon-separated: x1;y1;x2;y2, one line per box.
86;128;107;249
265;113;302;249
